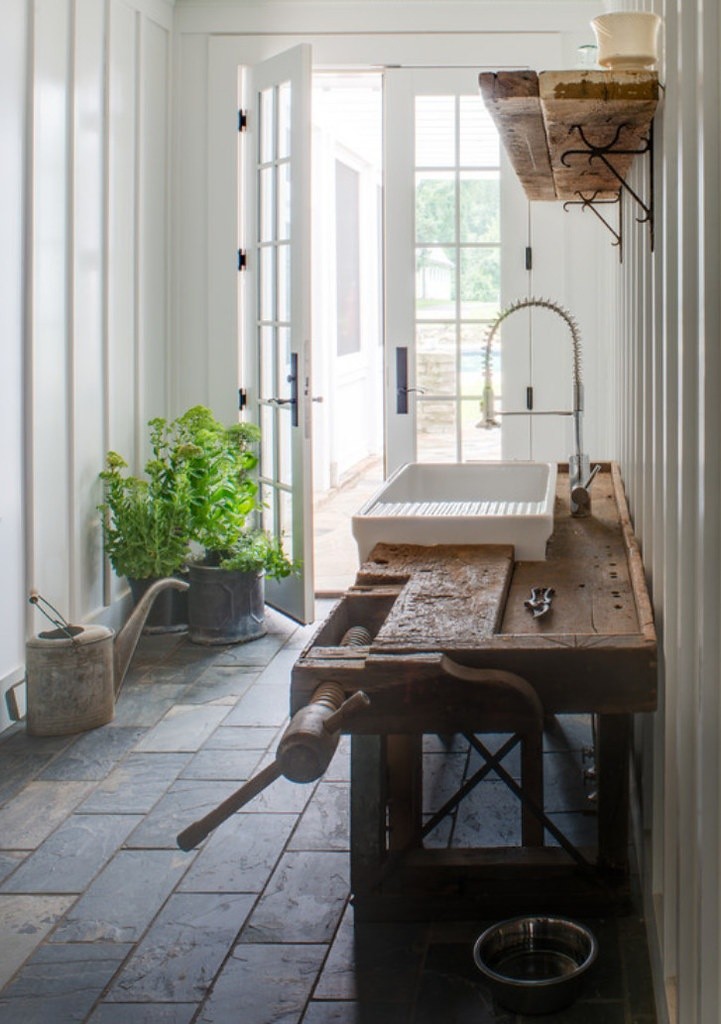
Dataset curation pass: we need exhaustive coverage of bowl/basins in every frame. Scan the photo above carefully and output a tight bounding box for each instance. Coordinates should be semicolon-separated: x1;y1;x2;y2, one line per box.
474;914;598;1014
591;10;665;71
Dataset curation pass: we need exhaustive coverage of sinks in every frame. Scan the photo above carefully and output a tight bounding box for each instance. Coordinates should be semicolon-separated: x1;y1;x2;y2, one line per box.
352;460;560;566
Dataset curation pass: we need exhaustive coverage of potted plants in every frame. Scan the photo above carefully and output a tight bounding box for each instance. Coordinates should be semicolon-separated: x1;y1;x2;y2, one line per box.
93;404;223;633
182;421;303;644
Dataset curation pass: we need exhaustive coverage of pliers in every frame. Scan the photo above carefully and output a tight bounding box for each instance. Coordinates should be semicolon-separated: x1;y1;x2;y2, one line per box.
523;586;555;620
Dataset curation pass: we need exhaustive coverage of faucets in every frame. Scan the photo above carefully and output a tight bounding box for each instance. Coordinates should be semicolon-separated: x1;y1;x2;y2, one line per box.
477;299;601;519
